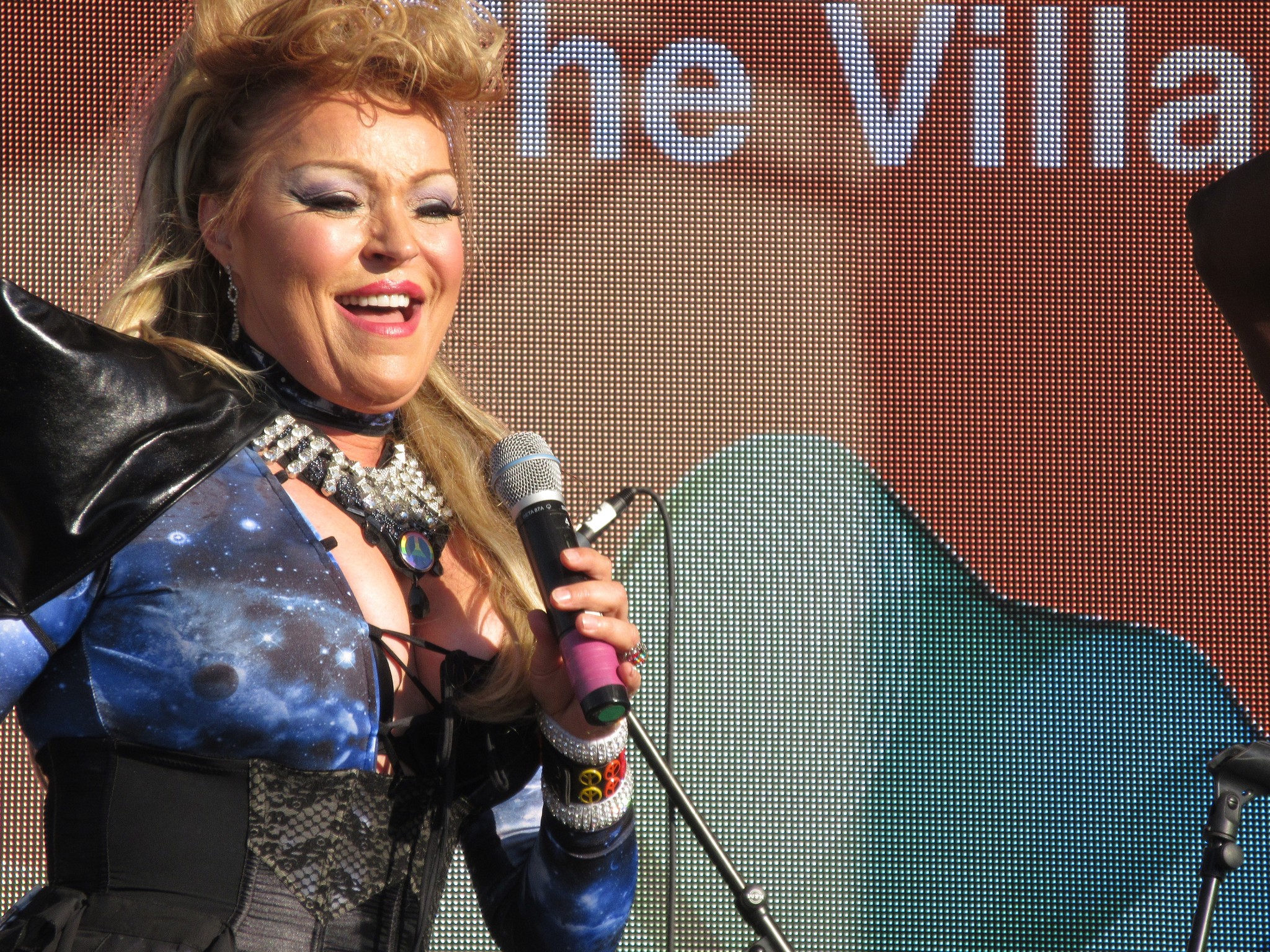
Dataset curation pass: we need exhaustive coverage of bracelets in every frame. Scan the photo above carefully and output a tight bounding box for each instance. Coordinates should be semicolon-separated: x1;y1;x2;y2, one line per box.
536;704;629;768
541;773;632;831
540;731;630;806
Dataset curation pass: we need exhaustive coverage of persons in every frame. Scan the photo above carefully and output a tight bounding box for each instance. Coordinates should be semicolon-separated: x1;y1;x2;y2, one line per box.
0;0;642;952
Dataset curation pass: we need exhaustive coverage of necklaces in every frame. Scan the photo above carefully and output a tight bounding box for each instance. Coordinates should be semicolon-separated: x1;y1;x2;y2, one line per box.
241;408;462;622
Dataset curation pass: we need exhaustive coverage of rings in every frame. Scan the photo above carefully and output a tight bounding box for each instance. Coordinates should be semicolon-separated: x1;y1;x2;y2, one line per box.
615;638;649;668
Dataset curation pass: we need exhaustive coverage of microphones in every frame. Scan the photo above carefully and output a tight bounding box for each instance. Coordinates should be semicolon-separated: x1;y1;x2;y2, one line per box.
492;428;631;727
